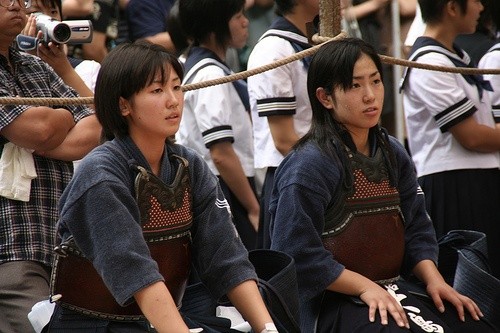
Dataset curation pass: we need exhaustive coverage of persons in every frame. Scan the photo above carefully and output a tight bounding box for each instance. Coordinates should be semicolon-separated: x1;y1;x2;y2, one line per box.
269;38;495;333
19;0;101;172
246;0;320;249
61;0;500;121
399;0;500;280
42;41;277;333
176;0;261;248
0;0;101;333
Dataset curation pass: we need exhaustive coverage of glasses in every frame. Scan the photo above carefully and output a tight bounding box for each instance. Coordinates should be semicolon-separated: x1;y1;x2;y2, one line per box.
0;0;31;8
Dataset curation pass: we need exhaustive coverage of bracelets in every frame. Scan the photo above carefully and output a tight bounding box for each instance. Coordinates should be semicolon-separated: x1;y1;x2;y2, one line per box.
262;322;278;333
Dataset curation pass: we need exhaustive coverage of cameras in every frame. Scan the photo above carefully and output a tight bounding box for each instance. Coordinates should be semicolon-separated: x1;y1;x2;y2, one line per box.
30;12;93;48
